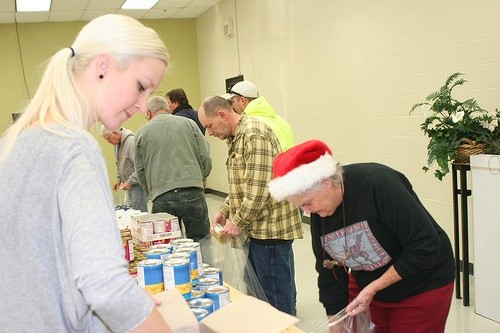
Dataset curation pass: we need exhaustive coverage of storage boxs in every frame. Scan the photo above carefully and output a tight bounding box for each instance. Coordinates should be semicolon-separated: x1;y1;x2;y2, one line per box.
129;230;182;243
152;287;300;333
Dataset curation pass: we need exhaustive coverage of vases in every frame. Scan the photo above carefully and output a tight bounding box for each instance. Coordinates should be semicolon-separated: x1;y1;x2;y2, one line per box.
450;144;487;165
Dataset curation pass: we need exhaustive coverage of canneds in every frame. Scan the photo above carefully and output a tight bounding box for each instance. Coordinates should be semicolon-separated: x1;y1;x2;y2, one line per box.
119;212;229;322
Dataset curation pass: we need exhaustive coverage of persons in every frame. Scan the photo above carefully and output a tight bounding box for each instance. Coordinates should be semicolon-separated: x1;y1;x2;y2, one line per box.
198;96;303;315
222;82;296;150
1;13;170;333
134;96;213;245
268;140;455;333
165;88;206;137
100;124;148;213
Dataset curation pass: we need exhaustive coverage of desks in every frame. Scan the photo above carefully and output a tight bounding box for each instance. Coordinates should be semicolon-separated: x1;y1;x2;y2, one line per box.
452;162;475;307
221;282;306;333
468;153;500;323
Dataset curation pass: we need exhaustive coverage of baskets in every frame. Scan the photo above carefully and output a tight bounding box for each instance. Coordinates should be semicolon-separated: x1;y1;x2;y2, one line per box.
453;137;484;163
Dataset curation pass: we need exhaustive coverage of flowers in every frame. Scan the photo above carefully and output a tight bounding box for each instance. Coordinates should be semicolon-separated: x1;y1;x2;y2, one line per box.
408;73;500;182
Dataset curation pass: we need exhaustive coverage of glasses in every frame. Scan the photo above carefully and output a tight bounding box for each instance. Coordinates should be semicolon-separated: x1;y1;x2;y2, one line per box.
227;82;244;98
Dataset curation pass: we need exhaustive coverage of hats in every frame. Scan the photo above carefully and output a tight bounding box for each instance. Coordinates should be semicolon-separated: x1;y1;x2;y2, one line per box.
221;78;259;103
268;140;337;202
